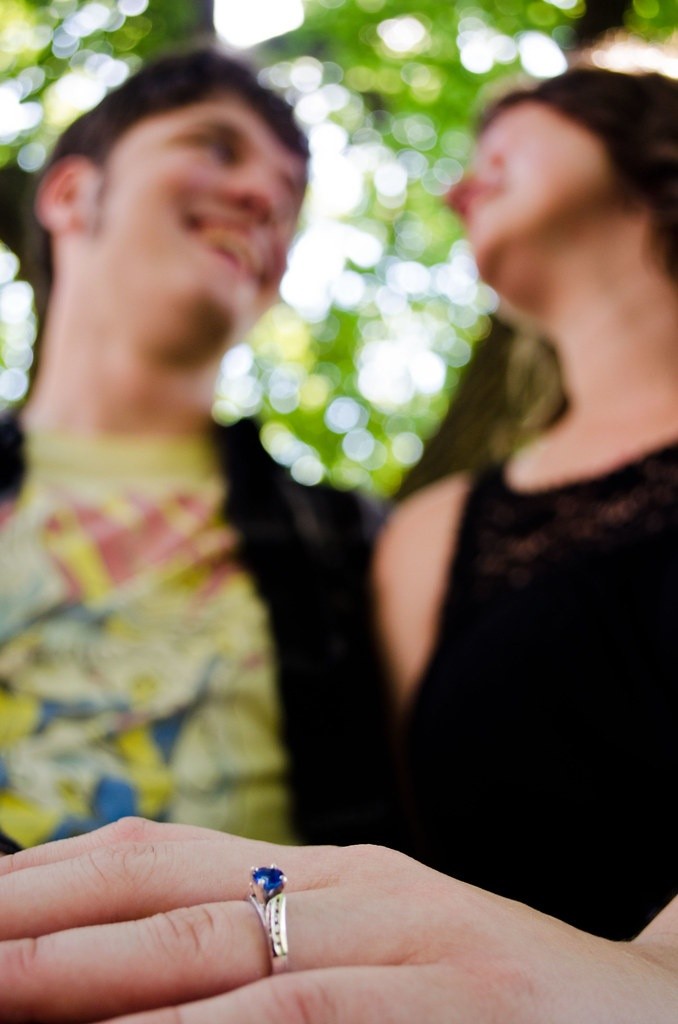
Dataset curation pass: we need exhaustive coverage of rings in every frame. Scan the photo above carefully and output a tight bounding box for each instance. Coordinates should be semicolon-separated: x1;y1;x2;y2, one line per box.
245;863;289;974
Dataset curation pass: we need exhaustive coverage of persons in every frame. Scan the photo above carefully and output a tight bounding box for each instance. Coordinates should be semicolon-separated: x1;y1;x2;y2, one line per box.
1;63;677;1024
0;47;401;862
